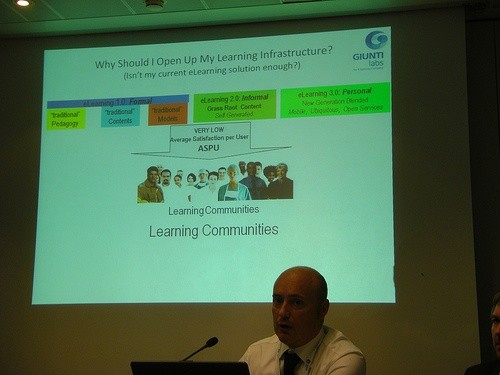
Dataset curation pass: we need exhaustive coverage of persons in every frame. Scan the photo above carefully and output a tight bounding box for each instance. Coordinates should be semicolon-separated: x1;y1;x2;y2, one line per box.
137;161;293;203
239;265;366;375
463;289;500;375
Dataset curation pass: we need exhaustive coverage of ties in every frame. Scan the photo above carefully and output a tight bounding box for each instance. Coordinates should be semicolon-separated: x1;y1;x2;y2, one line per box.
282;351;301;375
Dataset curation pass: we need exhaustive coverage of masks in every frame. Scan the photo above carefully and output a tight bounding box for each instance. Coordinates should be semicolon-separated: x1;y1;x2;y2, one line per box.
224;184;239;199
198;182;207;186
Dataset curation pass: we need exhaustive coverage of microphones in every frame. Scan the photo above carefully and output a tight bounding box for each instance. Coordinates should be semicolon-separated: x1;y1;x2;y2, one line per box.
181;337;218;361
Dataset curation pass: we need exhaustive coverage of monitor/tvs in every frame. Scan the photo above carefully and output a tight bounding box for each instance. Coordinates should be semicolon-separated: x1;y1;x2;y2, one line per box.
131;361;250;375
